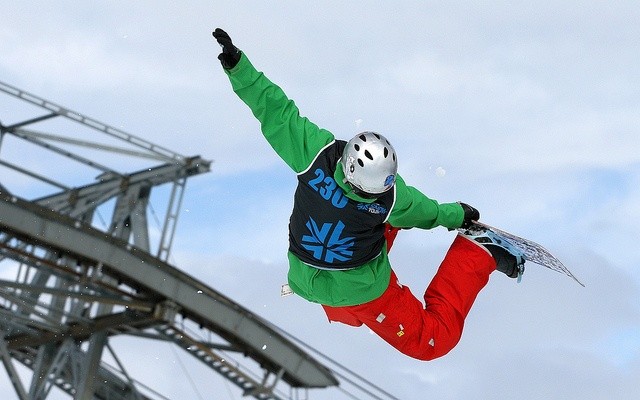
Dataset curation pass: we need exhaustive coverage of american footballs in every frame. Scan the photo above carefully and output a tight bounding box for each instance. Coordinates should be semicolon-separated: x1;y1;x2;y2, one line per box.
455;219;585;289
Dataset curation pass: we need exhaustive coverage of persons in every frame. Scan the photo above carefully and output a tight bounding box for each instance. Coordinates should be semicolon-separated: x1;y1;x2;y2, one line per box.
211;27;525;360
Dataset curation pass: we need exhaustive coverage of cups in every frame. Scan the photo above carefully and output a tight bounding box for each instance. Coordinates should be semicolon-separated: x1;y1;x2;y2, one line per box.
348;184;390;200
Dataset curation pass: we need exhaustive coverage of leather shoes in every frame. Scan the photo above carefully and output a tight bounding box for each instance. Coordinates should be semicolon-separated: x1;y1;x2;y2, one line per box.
458;202;480;229
211;27;240;70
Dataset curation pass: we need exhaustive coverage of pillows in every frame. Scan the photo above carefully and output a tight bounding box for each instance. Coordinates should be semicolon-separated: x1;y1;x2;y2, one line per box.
340;130;398;194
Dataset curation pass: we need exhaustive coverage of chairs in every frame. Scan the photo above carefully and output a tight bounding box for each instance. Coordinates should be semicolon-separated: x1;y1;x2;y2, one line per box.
467;230;525;284
